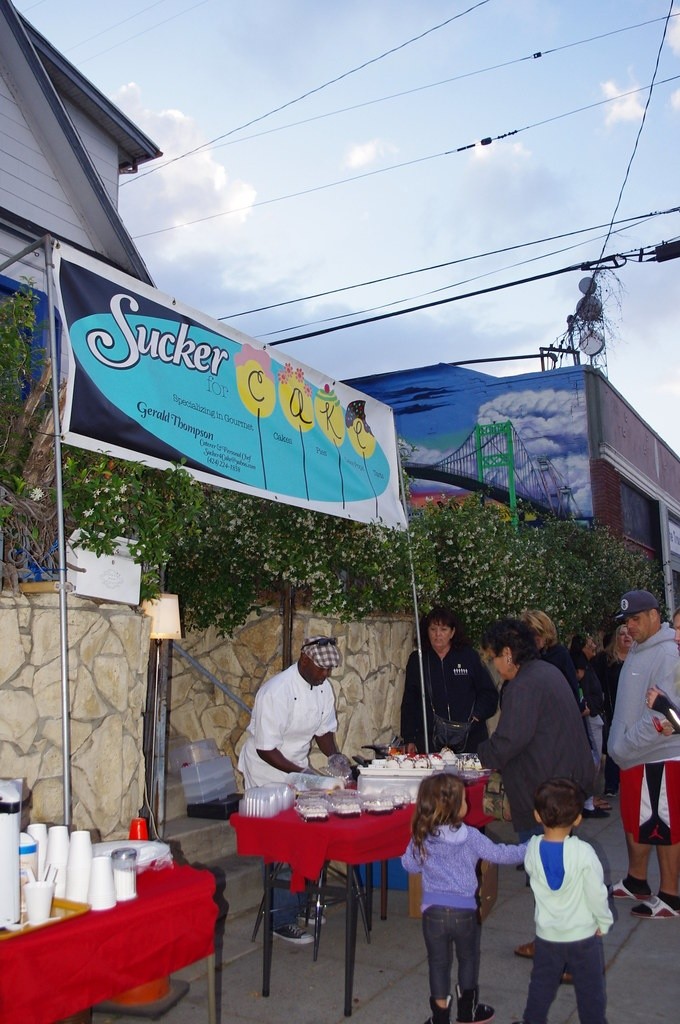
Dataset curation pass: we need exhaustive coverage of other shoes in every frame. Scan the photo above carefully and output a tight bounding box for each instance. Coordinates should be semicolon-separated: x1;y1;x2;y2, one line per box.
597;799;612;810
515;942;533;958
583;807;610;819
560;973;574;984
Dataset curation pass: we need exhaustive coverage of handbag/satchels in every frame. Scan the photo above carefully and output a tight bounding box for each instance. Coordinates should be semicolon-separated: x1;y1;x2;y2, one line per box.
433;715;471;752
483;772;512;822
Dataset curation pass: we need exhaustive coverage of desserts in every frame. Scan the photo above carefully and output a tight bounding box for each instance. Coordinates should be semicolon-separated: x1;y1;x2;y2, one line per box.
295;792;418;821
385;747;484;770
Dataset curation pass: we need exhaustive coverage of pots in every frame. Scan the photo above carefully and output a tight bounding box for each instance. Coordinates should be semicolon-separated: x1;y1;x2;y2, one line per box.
360;743;406;760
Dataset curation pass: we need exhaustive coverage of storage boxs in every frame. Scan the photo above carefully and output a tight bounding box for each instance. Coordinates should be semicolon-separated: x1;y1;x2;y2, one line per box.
187;792;245;822
67;528;141;605
171;737;221;773
180;758;236;795
353;852;411;889
409;851;497;922
183;770;238;804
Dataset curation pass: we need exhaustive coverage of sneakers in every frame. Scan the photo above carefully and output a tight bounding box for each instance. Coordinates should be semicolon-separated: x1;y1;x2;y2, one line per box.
297;908;327;925
273;923;315;943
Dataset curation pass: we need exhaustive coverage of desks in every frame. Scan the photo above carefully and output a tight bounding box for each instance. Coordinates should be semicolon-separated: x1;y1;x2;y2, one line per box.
0;861;229;1024
231;780;492;1016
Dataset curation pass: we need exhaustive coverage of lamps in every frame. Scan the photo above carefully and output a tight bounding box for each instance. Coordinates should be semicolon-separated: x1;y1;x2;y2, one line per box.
142;594;183;644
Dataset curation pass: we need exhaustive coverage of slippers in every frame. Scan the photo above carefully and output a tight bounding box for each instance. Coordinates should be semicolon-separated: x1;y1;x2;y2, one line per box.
630;895;680;919
609;880;654;899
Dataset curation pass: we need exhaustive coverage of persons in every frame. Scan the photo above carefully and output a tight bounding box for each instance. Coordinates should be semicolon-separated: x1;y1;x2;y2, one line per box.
238;634;351;945
607;590;680;918
474;619;600;984
500;608;680;817
399;608;498;754
513;779;614;1024
401;773;532;1024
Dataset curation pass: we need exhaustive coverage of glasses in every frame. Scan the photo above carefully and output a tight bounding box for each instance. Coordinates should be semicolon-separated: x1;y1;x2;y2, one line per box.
301;638;337;650
486;655;498;665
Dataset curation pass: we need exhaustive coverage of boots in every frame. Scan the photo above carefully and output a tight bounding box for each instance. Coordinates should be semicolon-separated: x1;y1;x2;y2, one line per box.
455;983;495;1024
424;994;453;1024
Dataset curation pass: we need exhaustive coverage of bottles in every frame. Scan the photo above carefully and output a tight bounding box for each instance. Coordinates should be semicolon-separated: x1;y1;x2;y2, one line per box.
111;848;138;902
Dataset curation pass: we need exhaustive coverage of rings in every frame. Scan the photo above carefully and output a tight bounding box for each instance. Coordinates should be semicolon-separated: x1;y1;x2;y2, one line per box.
645;698;648;703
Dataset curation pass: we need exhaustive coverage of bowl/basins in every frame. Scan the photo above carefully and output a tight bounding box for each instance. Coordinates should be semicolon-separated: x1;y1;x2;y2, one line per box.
92;839;170;874
237;782;413;822
369;759;456;770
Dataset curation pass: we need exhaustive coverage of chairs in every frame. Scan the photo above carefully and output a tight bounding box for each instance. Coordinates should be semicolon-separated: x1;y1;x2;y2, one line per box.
252;861;371;959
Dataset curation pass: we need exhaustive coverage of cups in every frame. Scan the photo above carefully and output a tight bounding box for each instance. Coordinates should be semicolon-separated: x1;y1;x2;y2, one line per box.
19;824;118;926
128;817;148;841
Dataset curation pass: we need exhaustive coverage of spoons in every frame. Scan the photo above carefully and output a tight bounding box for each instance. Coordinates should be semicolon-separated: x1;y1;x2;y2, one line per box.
2;916;63;930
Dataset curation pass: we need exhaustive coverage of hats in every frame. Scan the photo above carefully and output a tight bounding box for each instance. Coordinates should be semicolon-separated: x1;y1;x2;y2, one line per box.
614;590;659;619
302;635;339;669
